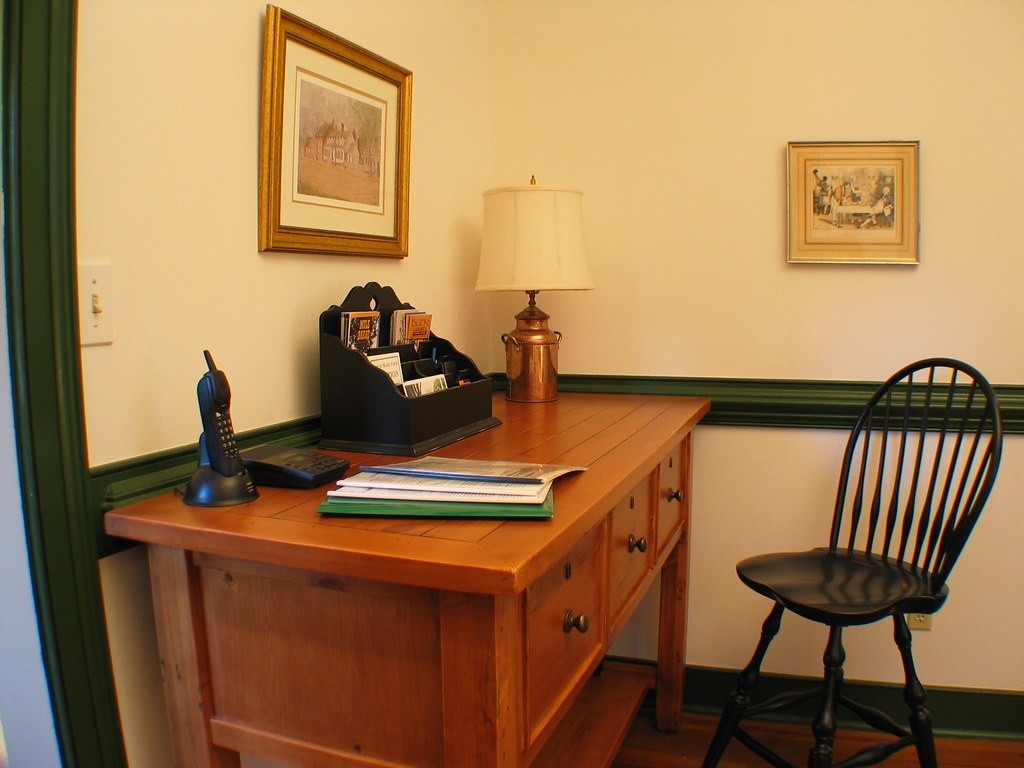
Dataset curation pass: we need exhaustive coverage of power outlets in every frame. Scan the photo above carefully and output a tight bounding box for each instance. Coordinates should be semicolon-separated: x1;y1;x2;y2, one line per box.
907;613;931;631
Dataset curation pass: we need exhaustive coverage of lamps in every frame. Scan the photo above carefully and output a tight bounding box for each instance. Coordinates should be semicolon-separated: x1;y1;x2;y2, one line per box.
476;173;596;402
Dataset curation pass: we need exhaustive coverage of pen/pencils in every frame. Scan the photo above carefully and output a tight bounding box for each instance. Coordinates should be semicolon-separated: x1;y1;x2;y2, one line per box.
431;347;437;362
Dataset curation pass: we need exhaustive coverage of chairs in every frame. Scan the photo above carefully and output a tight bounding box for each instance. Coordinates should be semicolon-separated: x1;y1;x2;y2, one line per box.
702;357;1003;768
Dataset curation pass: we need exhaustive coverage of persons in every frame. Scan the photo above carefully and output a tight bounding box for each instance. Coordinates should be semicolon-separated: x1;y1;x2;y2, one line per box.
814;171;890;227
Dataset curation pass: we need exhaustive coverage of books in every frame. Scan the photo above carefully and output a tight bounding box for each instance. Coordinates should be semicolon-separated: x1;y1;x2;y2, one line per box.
341;306;449;397
315;457;589;517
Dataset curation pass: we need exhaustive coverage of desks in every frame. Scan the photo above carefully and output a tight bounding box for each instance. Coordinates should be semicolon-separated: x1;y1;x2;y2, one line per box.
104;394;711;768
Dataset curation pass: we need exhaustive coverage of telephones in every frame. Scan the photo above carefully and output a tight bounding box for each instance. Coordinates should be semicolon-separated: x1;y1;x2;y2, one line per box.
181;350;260;509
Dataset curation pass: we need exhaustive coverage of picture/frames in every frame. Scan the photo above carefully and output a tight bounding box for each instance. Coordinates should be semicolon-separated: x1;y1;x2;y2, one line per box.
786;138;921;266
258;3;413;261
